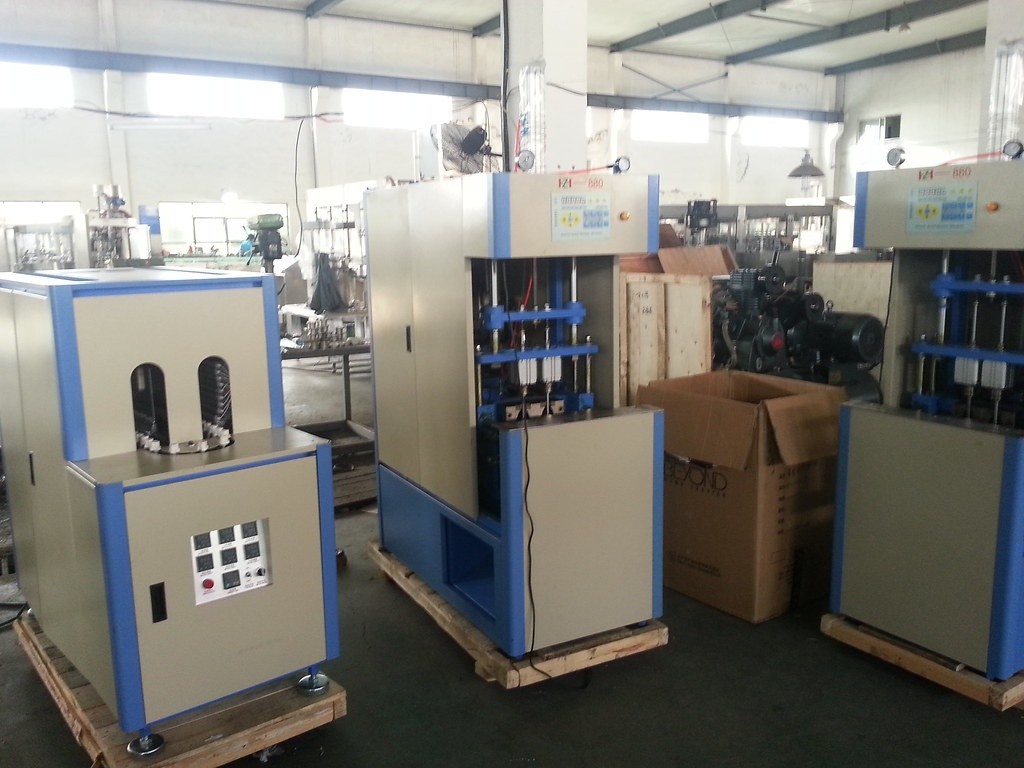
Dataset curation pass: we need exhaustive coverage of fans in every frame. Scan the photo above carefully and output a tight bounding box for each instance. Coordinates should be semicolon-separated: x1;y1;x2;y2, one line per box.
429;122;503;178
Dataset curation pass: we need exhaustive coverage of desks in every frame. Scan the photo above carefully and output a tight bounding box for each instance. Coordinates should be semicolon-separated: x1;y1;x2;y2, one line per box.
278;332;375;456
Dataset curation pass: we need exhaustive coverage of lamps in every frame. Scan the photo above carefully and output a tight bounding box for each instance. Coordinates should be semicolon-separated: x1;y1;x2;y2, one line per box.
788;150;823;194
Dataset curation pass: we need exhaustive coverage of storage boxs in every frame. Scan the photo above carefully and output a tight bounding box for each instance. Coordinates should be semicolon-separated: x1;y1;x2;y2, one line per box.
636;369;846;626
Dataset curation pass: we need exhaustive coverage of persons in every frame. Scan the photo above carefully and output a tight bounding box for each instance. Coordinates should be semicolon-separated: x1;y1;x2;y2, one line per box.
242;234;256;255
